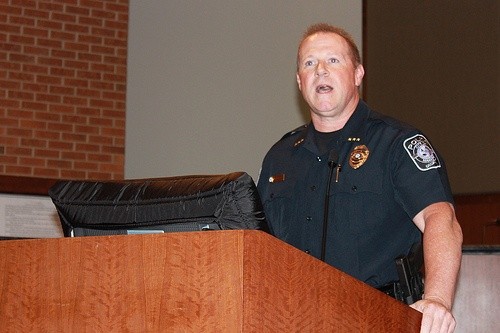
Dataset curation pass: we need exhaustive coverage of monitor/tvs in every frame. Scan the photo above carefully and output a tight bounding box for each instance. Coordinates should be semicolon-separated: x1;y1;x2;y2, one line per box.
49;172;276;238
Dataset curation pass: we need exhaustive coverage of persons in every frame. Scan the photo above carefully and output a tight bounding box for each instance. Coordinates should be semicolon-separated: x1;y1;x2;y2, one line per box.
256;24;463;333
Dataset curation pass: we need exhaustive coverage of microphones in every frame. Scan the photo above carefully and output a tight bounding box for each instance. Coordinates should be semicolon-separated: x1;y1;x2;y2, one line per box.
328;148;338;169
